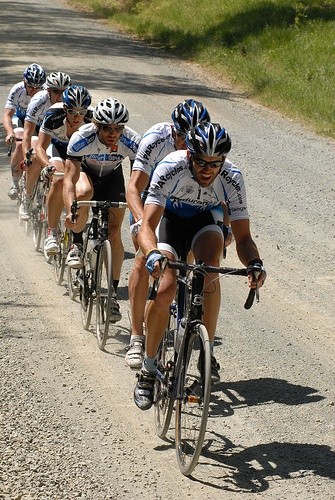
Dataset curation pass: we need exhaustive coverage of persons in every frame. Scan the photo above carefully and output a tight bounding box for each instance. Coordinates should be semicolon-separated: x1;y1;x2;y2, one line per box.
62;98;143;324
35;85;94;255
133;123;267;411
19;72;70;220
3;64;46;200
125;99;231;370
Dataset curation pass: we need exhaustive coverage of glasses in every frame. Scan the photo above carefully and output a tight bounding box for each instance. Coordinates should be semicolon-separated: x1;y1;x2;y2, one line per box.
96;122;125;133
64;104;87;116
192;154;225;167
24;81;43;91
173;126;187;138
49;89;63;95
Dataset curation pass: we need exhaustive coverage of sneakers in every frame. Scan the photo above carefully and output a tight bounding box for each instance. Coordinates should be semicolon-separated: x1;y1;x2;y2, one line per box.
134;366;156;409
198;354;221;381
19;197;33;220
103;296;123;321
124;336;144;367
66;242;83;268
8;183;19;197
44;235;59;252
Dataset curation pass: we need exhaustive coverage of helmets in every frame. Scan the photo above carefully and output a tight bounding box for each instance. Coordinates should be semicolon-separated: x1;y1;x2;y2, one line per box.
92;98;129;126
46;71;71;90
24;63;46;87
62;86;91;109
186;121;232;157
170;99;210;133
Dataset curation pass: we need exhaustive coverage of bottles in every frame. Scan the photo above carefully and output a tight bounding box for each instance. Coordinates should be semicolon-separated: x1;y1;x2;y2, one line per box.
175;316;187;354
90;245;99;270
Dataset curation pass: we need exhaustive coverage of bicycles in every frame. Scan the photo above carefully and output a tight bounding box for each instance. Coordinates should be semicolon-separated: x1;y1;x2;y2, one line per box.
6;137;129;349
123;223;263;475
